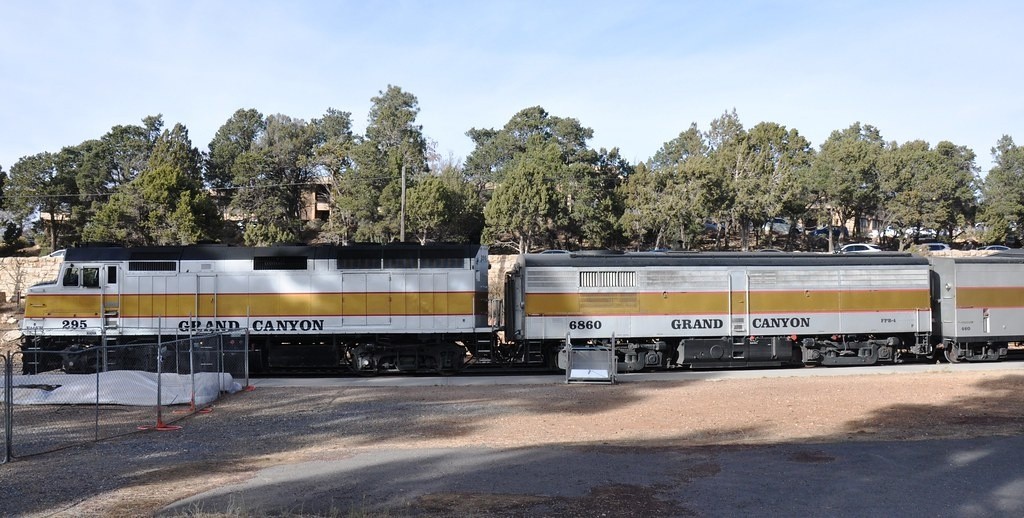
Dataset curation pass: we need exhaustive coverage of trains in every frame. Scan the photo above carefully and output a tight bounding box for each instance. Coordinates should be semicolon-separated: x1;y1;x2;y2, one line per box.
17;243;1023;376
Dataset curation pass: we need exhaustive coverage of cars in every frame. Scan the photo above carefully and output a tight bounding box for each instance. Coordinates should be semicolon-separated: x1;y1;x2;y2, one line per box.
977;245;1011;251
831;244;882;254
809;226;850;242
879;221;935;243
918;243;950;256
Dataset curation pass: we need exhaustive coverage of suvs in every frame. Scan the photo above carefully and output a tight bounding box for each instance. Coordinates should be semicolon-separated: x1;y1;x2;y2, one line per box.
758;218;790;242
